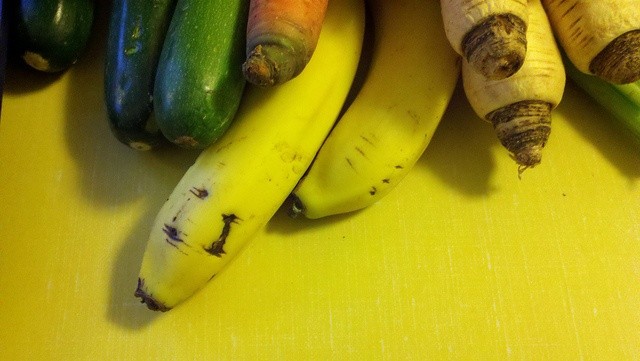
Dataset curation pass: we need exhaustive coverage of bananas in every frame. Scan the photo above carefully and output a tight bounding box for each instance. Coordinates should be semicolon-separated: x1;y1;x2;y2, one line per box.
290;2;463;217
134;1;369;312
